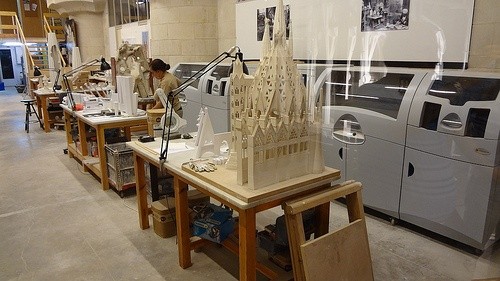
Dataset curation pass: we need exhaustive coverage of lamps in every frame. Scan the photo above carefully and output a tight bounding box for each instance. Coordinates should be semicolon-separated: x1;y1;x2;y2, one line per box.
159;46;249;175
62;57;112;112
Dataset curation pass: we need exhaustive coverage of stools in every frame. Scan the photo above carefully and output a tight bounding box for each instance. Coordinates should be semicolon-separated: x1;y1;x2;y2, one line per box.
21;99;45;133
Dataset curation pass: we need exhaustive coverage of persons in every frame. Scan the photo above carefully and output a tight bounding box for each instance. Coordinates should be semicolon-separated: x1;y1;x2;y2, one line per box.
149;58;184;119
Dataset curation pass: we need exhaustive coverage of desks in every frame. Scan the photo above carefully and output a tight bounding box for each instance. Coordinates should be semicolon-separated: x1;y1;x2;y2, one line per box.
59;103;148;191
125;132;341;281
32;90;61;132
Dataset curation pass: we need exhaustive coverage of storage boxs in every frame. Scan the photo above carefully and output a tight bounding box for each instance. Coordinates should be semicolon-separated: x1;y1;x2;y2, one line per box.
104;143;136;190
151;190;233;244
